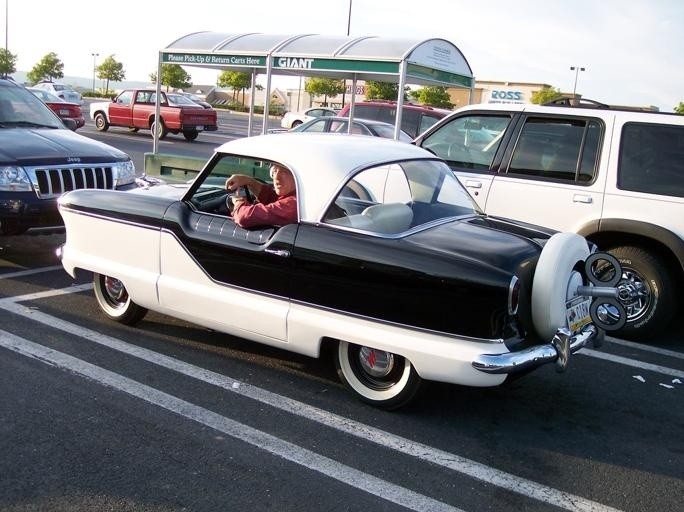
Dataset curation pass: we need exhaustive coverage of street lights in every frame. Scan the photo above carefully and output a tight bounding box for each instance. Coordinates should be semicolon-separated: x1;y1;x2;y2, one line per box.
566;63;587;100
90;51;101;97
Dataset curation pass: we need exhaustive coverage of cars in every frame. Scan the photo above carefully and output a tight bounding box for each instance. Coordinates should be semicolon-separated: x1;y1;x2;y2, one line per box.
0;73;220;241
50;127;635;418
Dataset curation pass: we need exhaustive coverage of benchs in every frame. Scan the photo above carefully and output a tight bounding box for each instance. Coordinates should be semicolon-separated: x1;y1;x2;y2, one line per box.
324;203;414;235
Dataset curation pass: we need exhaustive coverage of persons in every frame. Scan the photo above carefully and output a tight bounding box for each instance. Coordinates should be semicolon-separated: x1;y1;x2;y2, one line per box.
226;164;297;229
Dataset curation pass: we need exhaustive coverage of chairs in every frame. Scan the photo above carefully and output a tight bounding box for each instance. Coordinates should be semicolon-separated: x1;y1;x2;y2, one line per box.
511;137;576;171
